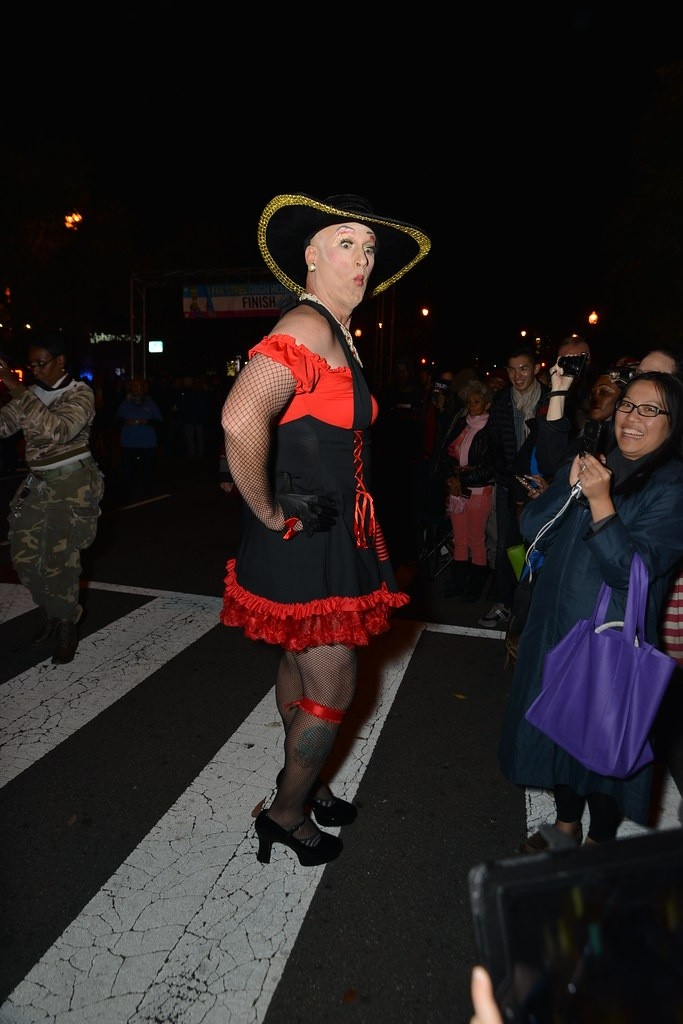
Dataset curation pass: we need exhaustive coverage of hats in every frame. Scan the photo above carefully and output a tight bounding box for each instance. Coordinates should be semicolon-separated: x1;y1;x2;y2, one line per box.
257;194;431;300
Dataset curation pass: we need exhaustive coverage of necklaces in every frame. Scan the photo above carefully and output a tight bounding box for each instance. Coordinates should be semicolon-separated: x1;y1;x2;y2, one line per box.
299;294;362;367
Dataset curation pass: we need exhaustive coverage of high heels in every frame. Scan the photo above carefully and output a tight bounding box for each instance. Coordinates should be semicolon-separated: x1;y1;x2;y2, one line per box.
254;810;344;866
276;766;358;826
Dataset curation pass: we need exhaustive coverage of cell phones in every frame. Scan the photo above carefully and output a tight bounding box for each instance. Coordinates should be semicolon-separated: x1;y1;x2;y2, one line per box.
515;472;541;492
472;827;682;1024
459;486;473;500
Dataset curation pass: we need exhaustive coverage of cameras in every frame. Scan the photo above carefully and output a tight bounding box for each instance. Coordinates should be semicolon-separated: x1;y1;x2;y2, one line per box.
558;352;589;379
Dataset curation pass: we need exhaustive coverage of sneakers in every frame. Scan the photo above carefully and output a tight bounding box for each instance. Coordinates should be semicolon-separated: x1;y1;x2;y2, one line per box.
478;603;512;627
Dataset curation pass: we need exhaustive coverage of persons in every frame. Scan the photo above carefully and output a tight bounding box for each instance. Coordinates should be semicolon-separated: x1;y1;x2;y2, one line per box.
0;336;241;664
218;192;434;866
376;321;683;852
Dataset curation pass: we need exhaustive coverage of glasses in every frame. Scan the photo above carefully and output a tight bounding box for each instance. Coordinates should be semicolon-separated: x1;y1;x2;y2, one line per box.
26;355;59;370
615;399;672;418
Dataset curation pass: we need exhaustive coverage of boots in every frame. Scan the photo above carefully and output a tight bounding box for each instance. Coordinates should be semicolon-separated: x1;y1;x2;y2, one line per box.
51;622;78;665
32;617;58;645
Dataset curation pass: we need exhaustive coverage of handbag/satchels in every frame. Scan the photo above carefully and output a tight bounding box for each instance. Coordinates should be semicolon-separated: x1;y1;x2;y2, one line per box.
523;551;674;776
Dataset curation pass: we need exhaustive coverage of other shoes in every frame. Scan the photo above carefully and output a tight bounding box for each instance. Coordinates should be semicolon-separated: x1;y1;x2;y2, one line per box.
437;580;466;600
460;582;484;604
523;821;585;855
507;616;519;643
